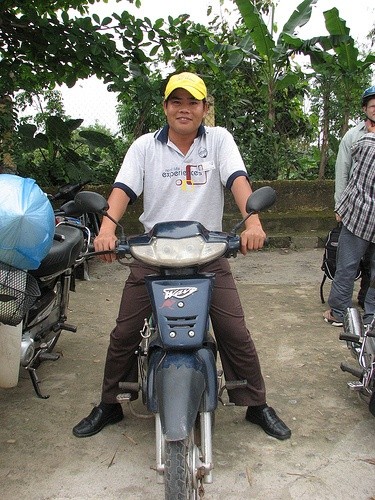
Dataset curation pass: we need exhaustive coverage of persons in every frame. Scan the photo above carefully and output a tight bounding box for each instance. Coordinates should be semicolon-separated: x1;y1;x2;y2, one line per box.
333;85;375;310
71;72;292;440
321;125;375;328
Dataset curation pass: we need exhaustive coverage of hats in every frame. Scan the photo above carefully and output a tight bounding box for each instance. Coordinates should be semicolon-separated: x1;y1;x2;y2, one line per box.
163;72;207;101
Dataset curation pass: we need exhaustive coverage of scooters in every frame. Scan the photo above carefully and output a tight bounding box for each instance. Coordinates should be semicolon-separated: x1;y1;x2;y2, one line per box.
75;186;277;500
0;200;91;398
339;307;375;416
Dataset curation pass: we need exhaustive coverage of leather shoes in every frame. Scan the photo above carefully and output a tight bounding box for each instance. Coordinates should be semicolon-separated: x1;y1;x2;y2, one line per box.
246;405;291;439
73;401;125;437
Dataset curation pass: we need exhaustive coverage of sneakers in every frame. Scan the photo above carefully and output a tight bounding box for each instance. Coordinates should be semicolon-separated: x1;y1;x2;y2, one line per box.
323;309;343;326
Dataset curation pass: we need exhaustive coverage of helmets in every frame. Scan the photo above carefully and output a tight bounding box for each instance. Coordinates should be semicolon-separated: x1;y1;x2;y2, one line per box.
361;86;375;97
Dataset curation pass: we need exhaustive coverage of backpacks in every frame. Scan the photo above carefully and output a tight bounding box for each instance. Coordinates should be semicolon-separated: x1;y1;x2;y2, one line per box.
321;221;365;280
